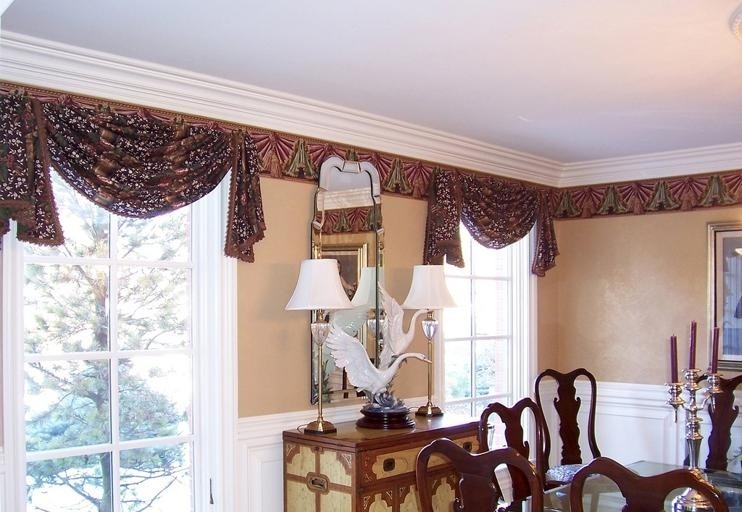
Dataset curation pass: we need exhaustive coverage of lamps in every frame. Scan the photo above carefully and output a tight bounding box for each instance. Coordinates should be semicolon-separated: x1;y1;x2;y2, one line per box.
283;257;458;434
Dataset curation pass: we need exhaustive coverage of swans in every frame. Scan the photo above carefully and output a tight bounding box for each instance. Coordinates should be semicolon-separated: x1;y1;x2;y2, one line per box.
326;322;433;407
376;280;433;369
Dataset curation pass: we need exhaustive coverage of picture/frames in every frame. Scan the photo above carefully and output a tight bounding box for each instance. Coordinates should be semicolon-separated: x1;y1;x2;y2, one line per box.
704;220;742;372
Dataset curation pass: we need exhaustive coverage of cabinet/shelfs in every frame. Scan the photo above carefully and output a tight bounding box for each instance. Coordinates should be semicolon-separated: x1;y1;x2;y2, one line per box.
282;409;496;511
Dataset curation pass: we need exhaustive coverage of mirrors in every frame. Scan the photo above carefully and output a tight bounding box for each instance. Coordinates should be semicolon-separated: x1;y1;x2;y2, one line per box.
309;154;385;407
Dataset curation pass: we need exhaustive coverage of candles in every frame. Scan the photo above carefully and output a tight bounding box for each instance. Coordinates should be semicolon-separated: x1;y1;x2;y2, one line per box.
669;320;720;383
